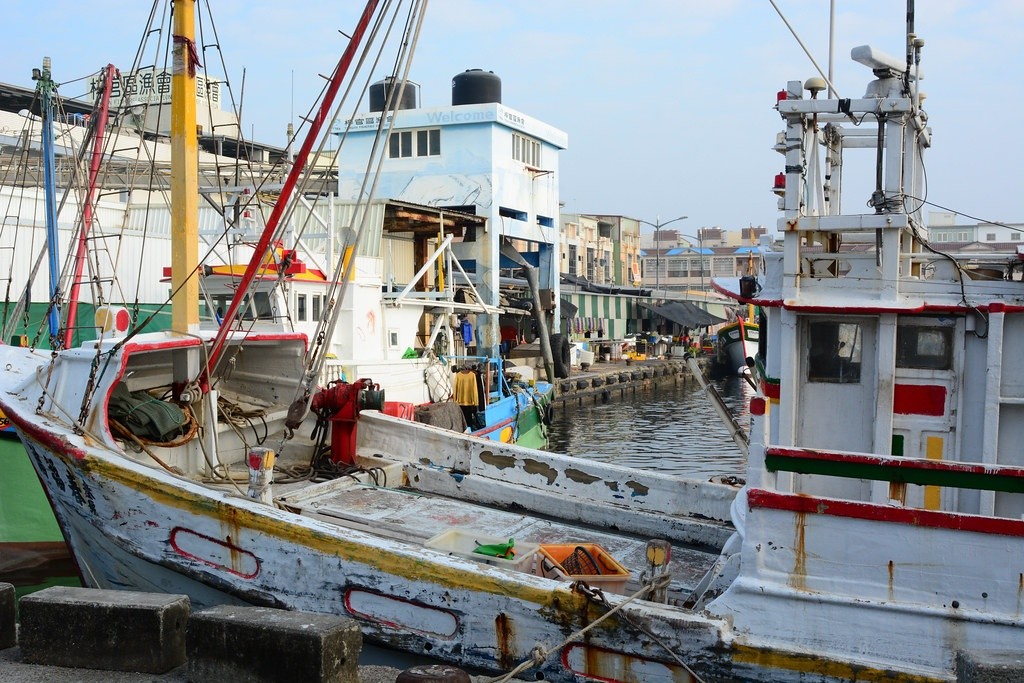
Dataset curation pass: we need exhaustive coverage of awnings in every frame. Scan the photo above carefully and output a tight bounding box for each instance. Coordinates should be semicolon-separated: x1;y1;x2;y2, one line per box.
637;301;727;330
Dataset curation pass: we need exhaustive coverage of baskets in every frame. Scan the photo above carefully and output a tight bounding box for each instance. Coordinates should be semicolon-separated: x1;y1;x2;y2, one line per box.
561;546;602;575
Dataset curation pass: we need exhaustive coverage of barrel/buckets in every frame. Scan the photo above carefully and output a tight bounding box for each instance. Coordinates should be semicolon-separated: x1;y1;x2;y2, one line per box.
580;362;591;372
604;353;611;361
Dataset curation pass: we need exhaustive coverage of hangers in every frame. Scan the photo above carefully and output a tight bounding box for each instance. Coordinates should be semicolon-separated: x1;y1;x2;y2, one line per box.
460;365;469;374
433;357;440;366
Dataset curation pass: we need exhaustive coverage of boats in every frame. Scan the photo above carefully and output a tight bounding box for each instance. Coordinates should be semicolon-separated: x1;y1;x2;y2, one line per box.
0;0;1024;682
360;353;557;456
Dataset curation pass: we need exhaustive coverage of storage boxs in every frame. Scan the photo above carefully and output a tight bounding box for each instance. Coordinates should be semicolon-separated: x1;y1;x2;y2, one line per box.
537;543;630;595
425;526;540;576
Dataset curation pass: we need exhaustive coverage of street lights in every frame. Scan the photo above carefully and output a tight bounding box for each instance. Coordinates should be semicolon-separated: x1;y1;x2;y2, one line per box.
674;228;728;292
634;215;689;290
